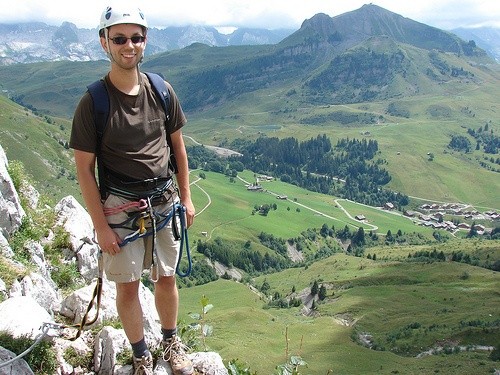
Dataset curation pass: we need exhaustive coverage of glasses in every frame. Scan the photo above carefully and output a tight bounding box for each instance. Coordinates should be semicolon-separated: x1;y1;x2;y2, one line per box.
108;35;145;44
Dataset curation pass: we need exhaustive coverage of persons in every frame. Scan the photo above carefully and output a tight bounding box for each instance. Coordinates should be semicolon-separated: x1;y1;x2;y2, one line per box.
68;4;196;375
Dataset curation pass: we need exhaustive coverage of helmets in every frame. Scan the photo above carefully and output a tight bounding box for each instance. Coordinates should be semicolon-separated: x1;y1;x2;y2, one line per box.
99;4;148;38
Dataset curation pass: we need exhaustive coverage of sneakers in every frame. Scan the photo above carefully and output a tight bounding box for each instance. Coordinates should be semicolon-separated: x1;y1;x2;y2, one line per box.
132;349;153;375
161;334;194;375
